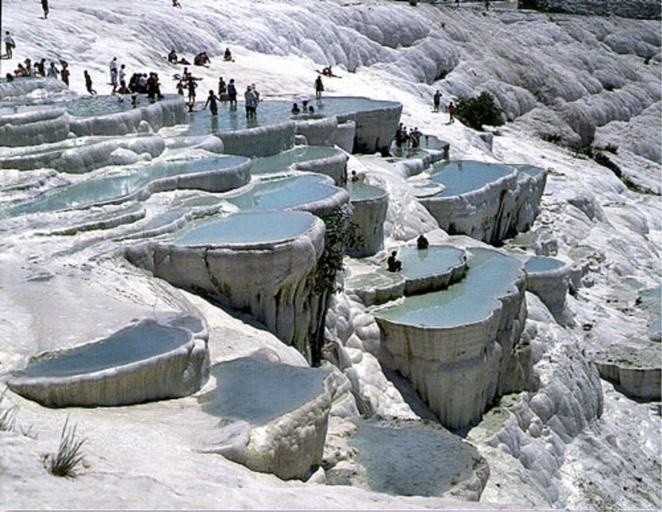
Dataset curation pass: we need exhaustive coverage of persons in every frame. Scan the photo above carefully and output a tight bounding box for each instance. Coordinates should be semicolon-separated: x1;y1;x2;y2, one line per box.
388;250;402;273
352;170;358;182
396;123;423;149
41;0;49;20
340;176;344;186
418;235;428;250
449;102;456;124
434;90;442;111
485;0;491;11
173;0;181;6
